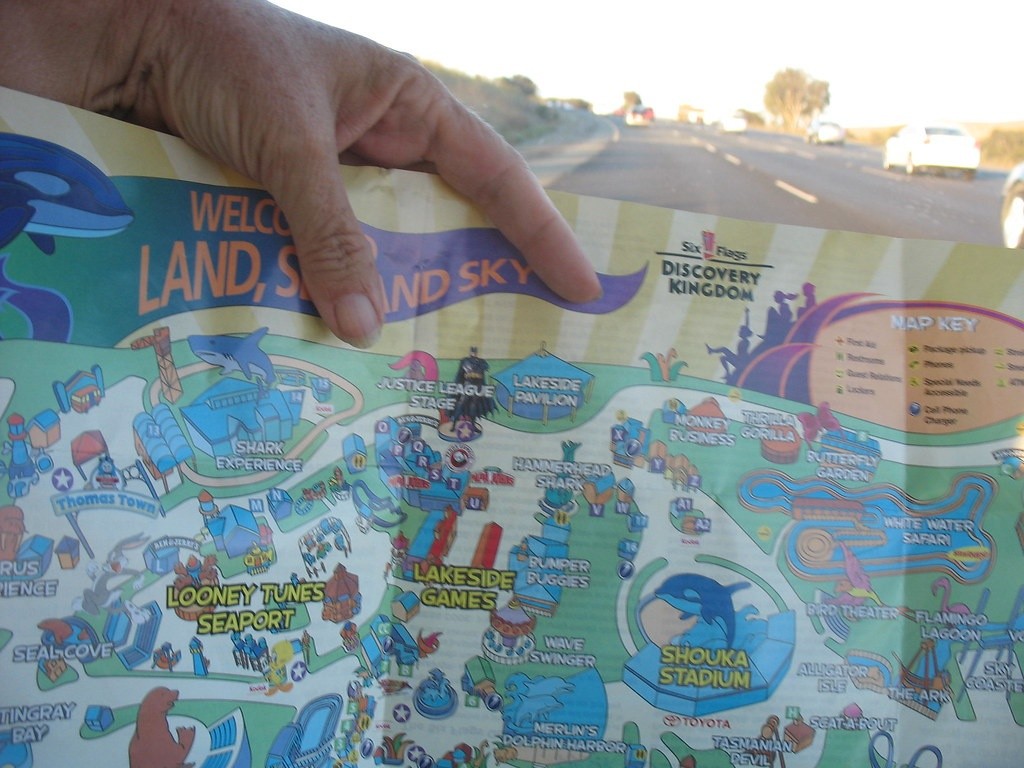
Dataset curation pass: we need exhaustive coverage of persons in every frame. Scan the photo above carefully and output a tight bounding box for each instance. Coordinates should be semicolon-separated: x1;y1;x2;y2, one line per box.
0;0;602;348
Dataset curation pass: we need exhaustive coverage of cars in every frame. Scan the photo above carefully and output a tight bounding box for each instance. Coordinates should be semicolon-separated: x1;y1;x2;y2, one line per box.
723;117;748;133
1002;163;1024;247
806;119;844;145
626;104;655;126
883;121;981;179
